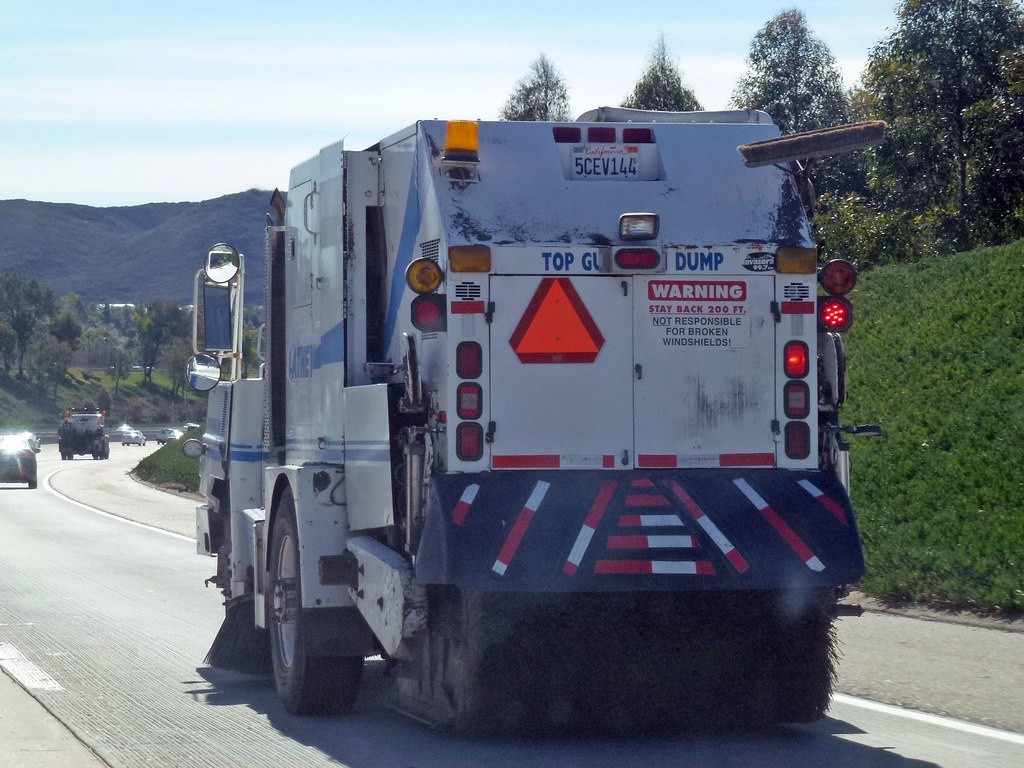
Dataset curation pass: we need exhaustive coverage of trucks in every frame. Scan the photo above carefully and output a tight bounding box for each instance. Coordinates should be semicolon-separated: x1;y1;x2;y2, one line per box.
180;104;885;739
58;406;109;461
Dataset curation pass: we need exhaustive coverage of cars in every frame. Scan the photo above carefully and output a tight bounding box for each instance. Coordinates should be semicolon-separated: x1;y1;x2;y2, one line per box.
0;435;41;489
156;428;183;445
122;430;146;446
17;431;41;453
